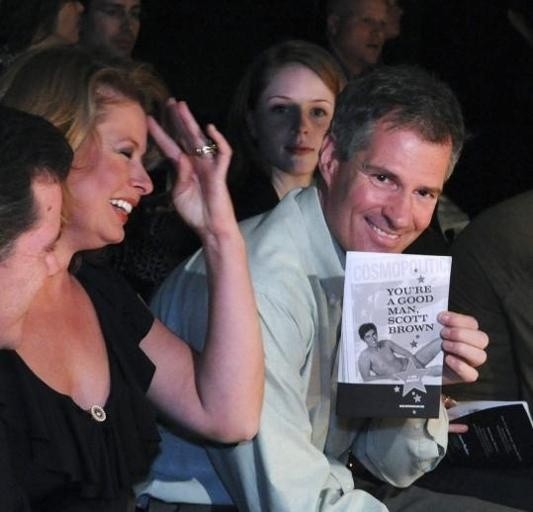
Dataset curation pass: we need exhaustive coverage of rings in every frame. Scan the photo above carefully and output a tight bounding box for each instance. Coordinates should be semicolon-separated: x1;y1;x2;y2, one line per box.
191;144;217;158
444;395;450;405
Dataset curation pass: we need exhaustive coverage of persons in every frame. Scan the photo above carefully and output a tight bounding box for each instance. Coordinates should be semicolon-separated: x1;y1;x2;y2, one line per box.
0;43;267;512
1;105;72;353
0;0;173;105
358;323;444;383
442;192;533;407
128;63;527;512
385;0;405;68
320;0;389;84
177;39;346;264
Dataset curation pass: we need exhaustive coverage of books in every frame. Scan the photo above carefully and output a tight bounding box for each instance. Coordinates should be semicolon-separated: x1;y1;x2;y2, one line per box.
445;399;533;473
335;251;452;419
